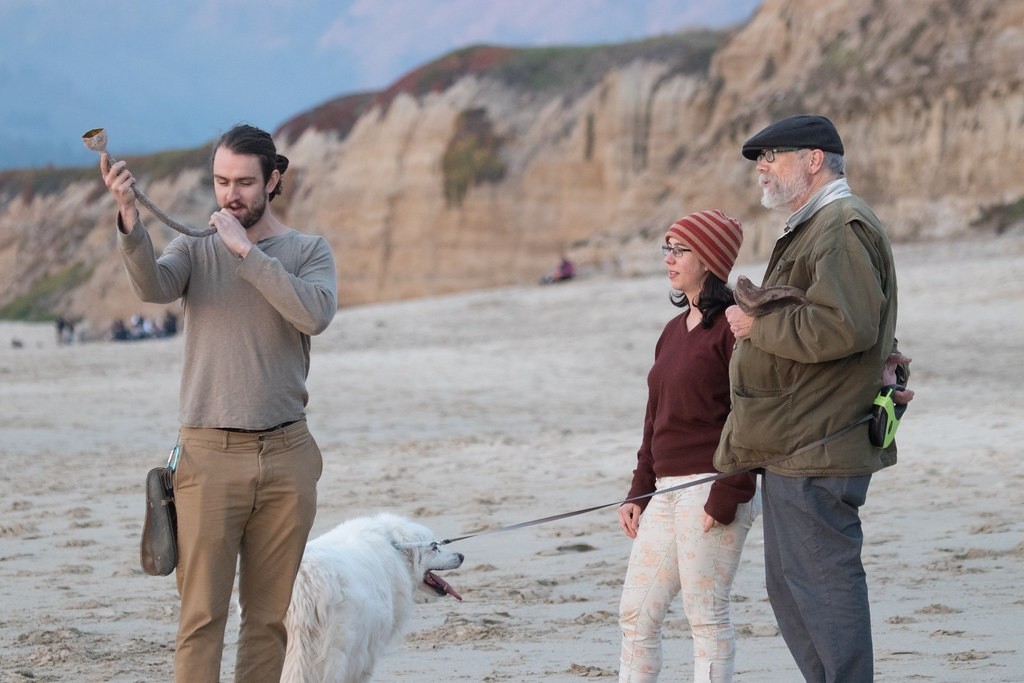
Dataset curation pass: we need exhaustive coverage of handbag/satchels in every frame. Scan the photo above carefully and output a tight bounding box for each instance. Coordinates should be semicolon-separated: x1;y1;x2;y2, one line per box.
138;465;177;578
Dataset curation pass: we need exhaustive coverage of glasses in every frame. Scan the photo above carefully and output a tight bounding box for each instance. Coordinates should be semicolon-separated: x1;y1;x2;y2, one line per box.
662;244;692;258
756;147;802;163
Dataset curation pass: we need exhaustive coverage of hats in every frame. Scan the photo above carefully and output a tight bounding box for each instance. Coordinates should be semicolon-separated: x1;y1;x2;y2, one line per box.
666;209;744;284
742;114;845;160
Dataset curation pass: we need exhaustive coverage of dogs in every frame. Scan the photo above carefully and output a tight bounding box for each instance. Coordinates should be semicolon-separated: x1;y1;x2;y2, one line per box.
236;512;464;683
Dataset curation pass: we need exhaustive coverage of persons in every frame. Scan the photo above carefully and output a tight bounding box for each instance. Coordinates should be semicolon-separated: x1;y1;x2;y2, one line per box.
712;115;914;683
101;124;338;683
57;306;178;343
550;256;573;280
619;208;760;683
